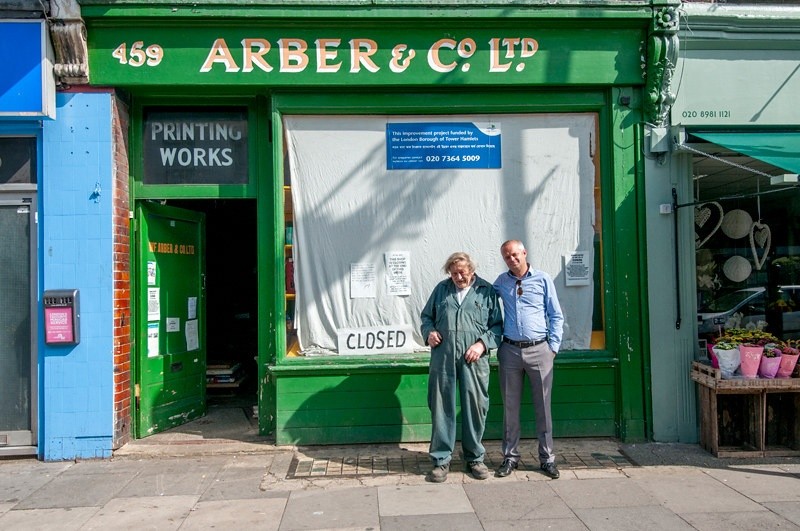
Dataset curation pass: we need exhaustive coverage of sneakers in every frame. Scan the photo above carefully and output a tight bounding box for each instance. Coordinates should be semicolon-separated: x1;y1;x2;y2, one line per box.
469;461;489;479
430;463;450;483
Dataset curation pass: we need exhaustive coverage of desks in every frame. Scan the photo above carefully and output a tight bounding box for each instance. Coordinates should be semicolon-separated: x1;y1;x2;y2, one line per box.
692;362;800;458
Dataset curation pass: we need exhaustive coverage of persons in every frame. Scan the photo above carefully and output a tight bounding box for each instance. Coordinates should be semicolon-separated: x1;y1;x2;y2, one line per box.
491;240;564;478
420;251;503;482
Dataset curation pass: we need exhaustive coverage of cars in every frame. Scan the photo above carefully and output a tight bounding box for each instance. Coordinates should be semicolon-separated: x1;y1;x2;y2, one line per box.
697;285;800;343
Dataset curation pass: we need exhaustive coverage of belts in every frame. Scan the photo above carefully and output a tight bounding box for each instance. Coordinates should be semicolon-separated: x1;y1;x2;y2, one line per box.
503;336;547;349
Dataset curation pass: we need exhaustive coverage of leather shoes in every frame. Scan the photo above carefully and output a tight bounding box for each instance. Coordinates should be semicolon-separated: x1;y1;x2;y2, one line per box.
495;459;518;477
540;462;559;478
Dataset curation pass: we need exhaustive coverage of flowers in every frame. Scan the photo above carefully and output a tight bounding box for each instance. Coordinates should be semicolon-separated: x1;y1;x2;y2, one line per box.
709;327;799;359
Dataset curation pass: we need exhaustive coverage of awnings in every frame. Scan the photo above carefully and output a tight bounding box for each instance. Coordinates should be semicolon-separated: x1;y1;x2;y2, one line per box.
686;126;800;175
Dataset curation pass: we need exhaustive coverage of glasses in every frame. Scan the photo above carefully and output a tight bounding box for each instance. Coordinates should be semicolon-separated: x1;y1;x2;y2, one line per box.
516;280;523;297
